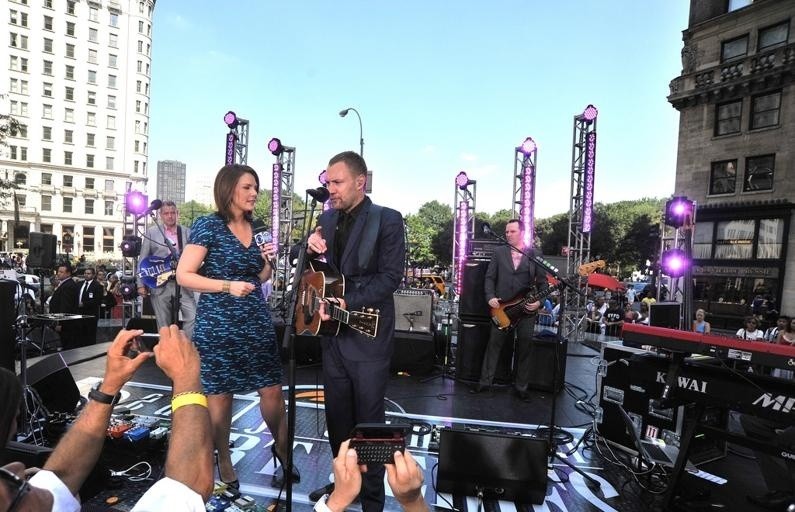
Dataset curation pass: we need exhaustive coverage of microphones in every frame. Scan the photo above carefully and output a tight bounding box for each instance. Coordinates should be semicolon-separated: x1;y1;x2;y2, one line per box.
307;186;330;202
481;220;492;234
137;199;163;222
252;218;278;271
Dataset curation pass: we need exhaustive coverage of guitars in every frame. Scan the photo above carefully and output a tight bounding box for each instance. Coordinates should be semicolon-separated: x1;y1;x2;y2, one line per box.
289;256;383;341
487;254;607;335
138;253;183;290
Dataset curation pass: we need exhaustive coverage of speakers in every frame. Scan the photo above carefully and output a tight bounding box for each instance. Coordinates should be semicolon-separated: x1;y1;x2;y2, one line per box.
454;322;490;383
458;260;493;321
29;232;56;266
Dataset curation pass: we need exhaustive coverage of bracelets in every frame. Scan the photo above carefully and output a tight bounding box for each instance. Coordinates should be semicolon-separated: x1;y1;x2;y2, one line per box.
171;391;208;411
221;279;230;293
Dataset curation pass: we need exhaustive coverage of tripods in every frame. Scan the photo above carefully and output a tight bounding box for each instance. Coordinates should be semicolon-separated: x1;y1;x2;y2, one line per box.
491;231;601;490
18;271;61;358
420;312;475;386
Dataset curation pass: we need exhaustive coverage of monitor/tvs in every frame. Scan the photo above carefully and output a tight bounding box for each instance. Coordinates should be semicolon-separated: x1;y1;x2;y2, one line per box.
142;293;157;319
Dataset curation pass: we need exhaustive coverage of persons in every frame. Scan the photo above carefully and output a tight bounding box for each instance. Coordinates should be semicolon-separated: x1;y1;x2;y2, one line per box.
290;151;405;512
765;315;792;344
137;203;198;342
776;319;795;345
176;164;300;493
736;316;764;343
692;309;711;336
1;325;214;512
538;268;663;335
0;253;125;323
469;219;549;403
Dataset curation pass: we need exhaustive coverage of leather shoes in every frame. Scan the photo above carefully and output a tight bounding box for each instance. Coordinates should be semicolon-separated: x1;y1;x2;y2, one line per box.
517;390;530;402
469;385;489;394
309;482;335;501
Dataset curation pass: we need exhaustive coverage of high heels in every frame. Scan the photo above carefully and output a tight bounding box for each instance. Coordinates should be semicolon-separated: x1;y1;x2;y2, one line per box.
271;442;299;482
216;455;240;489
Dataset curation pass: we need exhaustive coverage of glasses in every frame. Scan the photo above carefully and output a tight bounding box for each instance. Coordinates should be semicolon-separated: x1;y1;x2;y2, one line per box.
0;465;33;512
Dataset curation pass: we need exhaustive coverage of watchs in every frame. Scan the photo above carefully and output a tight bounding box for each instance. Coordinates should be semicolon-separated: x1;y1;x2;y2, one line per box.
87;381;121;405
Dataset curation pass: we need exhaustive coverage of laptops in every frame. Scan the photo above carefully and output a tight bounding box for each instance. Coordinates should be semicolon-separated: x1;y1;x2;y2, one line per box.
436;429;548;505
618;403;698;473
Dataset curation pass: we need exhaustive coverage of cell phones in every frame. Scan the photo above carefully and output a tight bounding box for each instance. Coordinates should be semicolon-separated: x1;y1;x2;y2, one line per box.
348;423;411;464
134;333;162;353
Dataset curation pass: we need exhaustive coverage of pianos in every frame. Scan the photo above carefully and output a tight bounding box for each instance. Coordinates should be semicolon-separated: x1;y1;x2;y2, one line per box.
623;354;795;427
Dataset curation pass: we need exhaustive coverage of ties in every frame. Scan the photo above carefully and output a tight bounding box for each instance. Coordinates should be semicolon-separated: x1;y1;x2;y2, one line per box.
81;281;88;302
